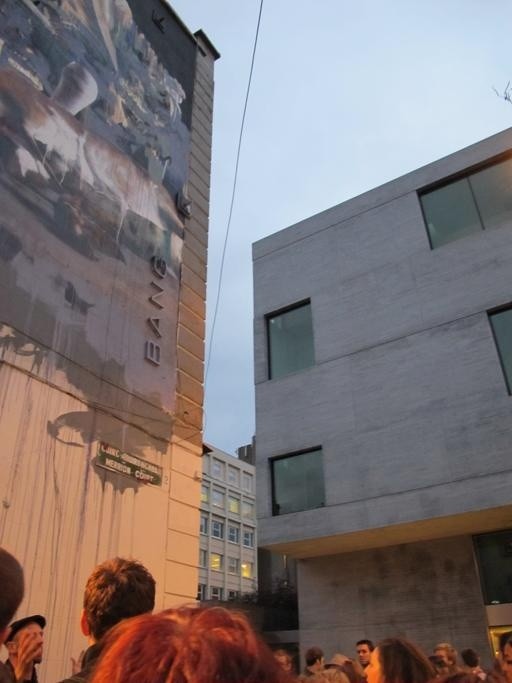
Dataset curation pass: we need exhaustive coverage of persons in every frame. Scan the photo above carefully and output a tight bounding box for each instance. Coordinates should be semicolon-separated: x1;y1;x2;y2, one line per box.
0;548;25;629
91;603;288;682
61;557;156;682
0;615;84;683
275;632;512;682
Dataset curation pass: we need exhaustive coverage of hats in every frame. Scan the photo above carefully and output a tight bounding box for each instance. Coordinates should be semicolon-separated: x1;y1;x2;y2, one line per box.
325;653;354;676
1;613;48;648
